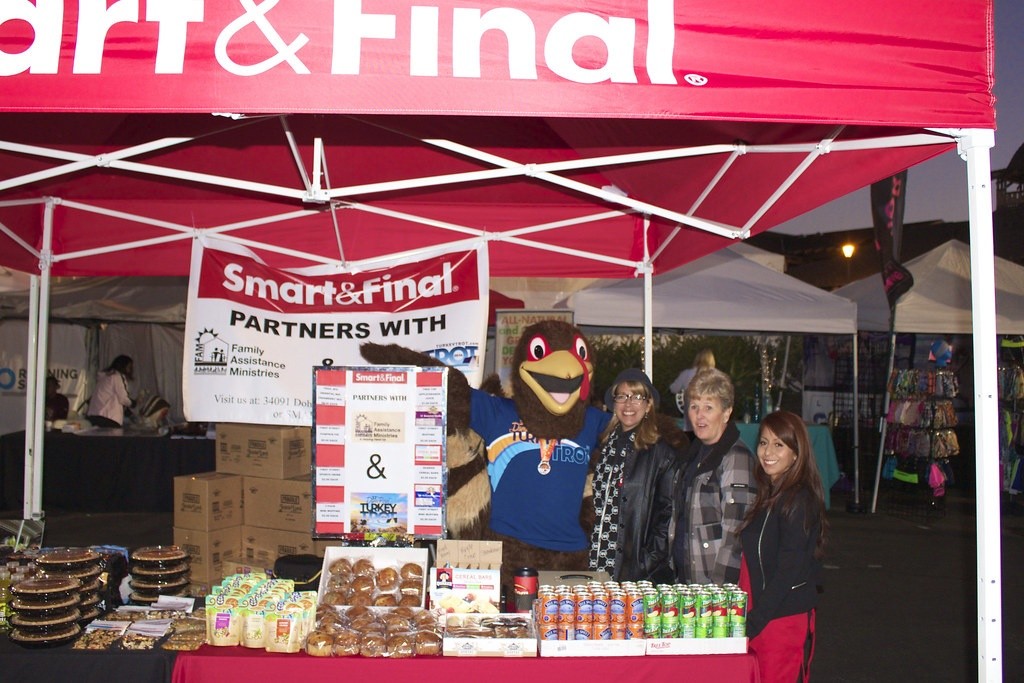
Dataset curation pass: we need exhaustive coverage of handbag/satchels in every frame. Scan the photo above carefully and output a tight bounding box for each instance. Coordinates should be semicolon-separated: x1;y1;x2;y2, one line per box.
270;553;325;592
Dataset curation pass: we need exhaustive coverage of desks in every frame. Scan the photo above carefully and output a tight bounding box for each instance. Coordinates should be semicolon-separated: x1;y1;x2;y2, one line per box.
173;643;756;682
26;421;213;512
675;420;839;509
0;595;203;683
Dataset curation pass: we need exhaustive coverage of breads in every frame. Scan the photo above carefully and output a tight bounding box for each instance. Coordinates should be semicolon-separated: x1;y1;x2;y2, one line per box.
446;616;532;638
304;559;442;657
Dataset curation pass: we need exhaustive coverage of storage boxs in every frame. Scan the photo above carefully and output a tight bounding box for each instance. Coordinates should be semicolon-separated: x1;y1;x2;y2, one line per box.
169;423;330;586
317;539;753;660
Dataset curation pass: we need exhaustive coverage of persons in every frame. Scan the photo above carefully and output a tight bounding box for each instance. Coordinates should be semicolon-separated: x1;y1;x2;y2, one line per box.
668;366;760;588
736;411;830;683
87;355;136;429
669;347;715;431
588;368;681;588
45;376;69;421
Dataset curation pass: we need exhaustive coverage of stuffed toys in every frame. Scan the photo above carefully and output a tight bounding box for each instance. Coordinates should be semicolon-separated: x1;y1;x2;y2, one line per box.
360;320;690;601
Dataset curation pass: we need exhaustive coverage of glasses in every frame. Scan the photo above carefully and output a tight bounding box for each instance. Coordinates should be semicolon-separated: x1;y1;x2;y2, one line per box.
612;393;647;406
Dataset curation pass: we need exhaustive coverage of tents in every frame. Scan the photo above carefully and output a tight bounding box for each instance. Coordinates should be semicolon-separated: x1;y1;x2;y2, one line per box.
0;0;1003;683
0;276;188;323
832;239;1024;512
553;248;859;503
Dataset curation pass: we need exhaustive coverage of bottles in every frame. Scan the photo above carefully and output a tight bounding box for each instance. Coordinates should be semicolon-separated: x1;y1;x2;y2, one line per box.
0;562;36;633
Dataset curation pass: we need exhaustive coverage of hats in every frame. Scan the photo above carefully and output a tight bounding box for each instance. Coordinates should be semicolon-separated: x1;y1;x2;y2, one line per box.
604;368;660;411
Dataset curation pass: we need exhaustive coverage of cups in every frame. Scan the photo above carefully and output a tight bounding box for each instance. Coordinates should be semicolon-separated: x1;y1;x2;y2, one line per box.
514;567;538;614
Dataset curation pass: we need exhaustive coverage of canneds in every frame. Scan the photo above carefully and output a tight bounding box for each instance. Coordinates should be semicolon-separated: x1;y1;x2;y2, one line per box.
535;580;748;640
